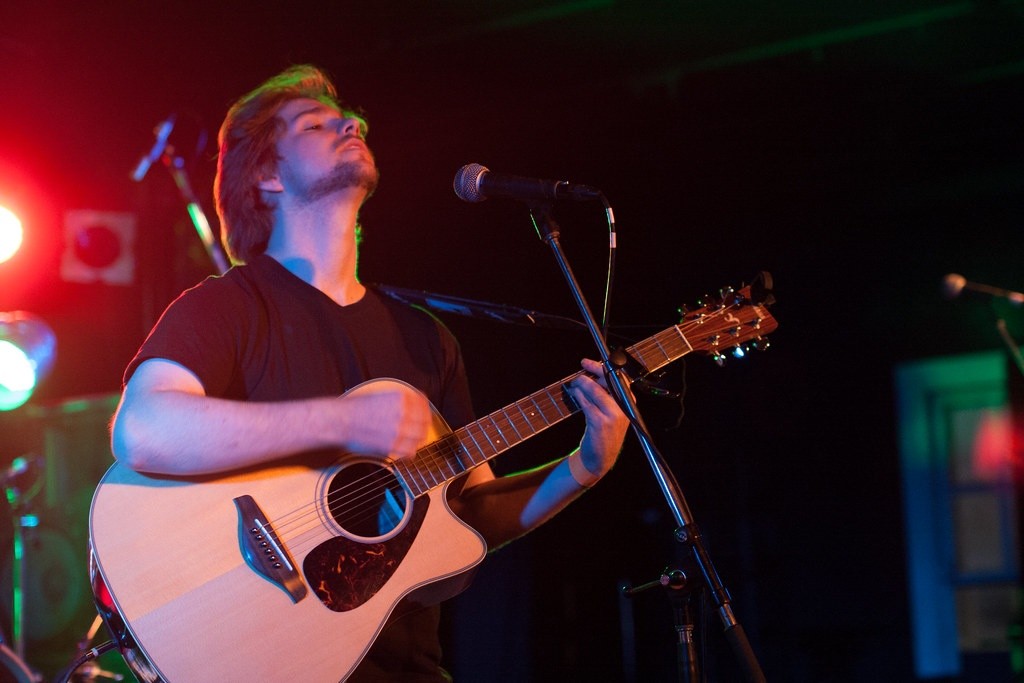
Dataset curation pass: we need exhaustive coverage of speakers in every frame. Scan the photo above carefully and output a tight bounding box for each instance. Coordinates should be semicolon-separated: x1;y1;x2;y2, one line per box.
0;394;127;683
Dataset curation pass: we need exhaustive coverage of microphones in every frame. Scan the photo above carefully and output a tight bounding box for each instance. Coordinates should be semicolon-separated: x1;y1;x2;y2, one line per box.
0;451;34;483
944;274;1024;307
453;164;601;203
131;116;175;182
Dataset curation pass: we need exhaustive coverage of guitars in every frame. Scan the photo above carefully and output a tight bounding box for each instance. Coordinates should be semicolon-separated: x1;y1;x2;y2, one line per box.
87;267;777;680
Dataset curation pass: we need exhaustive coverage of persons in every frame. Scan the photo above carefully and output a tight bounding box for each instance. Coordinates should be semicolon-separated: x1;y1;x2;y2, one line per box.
112;66;638;683
